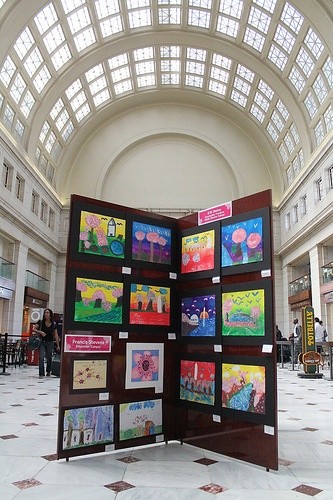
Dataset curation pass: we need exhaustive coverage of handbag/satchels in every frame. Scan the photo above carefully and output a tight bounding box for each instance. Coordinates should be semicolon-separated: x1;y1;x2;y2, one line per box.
27;336;42;351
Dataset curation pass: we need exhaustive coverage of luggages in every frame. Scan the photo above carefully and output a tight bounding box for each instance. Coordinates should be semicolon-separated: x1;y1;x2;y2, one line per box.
51;350;60;376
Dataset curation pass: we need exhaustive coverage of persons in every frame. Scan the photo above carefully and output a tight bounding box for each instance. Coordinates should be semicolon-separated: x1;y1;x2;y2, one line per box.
292;318;302;364
314;317;324;353
319;320;327;341
276;325;281;346
33;309;60;378
289;332;293;341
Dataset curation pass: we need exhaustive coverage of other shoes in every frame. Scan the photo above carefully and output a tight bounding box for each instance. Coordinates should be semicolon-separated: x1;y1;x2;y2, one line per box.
46;372;50;376
40;375;43;379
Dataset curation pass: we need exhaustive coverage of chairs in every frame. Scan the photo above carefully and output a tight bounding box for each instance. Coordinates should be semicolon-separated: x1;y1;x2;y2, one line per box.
0;339;28;368
